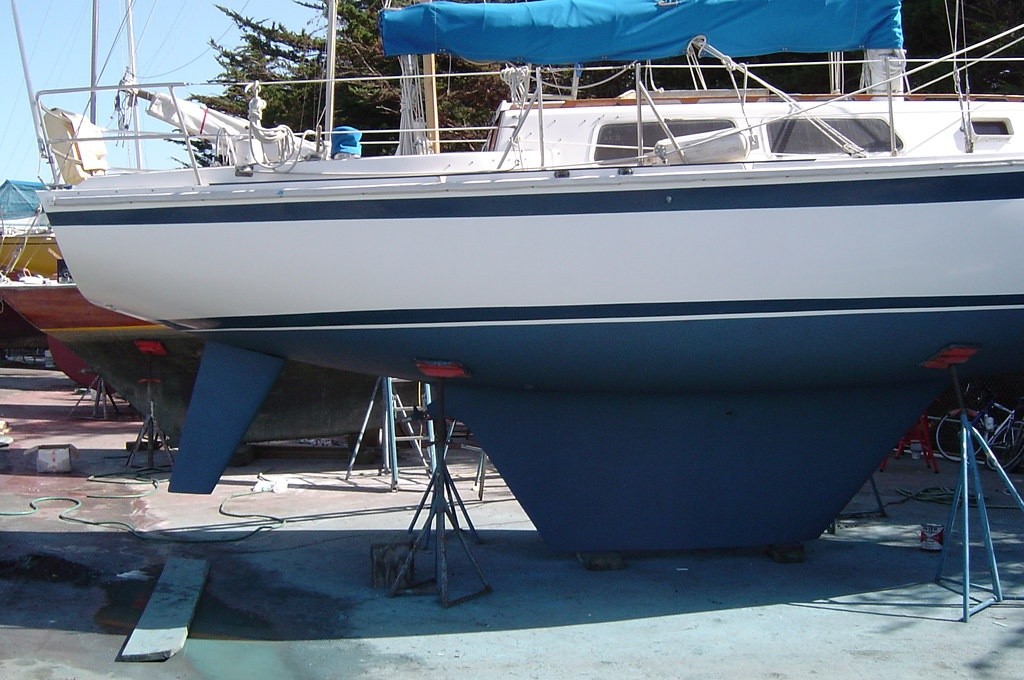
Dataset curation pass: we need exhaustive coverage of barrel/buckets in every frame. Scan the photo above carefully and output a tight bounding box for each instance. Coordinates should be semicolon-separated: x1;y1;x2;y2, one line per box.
91;388;108;400
921;523;944;550
911;440;922;459
45;350;55;367
272;475;287;492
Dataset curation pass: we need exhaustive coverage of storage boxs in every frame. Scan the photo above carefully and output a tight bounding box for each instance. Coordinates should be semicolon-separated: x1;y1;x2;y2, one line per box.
23;443;80;473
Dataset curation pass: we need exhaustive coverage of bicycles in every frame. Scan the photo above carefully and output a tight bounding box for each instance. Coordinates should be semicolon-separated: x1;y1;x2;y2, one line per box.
935;379;1023;474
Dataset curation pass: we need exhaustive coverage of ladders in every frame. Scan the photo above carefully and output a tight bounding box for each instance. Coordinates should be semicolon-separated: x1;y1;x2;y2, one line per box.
379;376;442;487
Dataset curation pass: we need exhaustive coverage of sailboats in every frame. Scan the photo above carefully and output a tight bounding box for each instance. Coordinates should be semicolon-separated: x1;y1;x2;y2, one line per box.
0;0;1024;554
